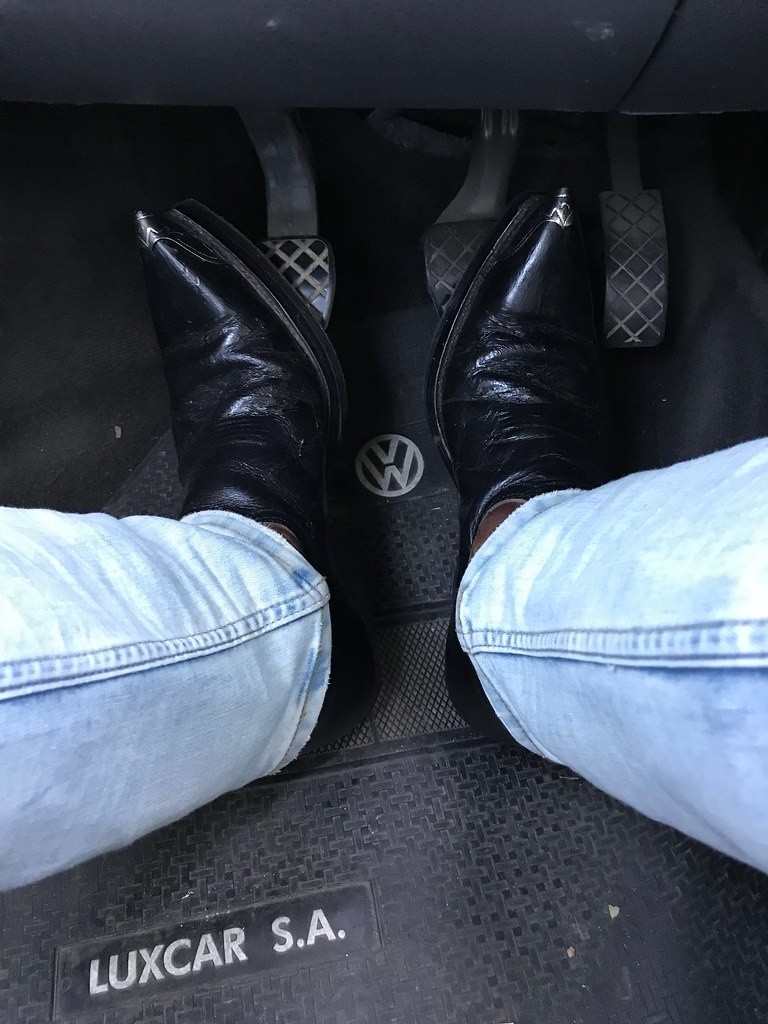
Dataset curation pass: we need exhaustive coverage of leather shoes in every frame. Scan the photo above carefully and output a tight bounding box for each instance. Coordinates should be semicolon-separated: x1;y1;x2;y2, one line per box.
422;179;611;736
131;197;377;747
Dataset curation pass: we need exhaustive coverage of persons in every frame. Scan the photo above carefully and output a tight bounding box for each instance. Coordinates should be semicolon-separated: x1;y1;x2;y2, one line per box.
0;177;768;895
